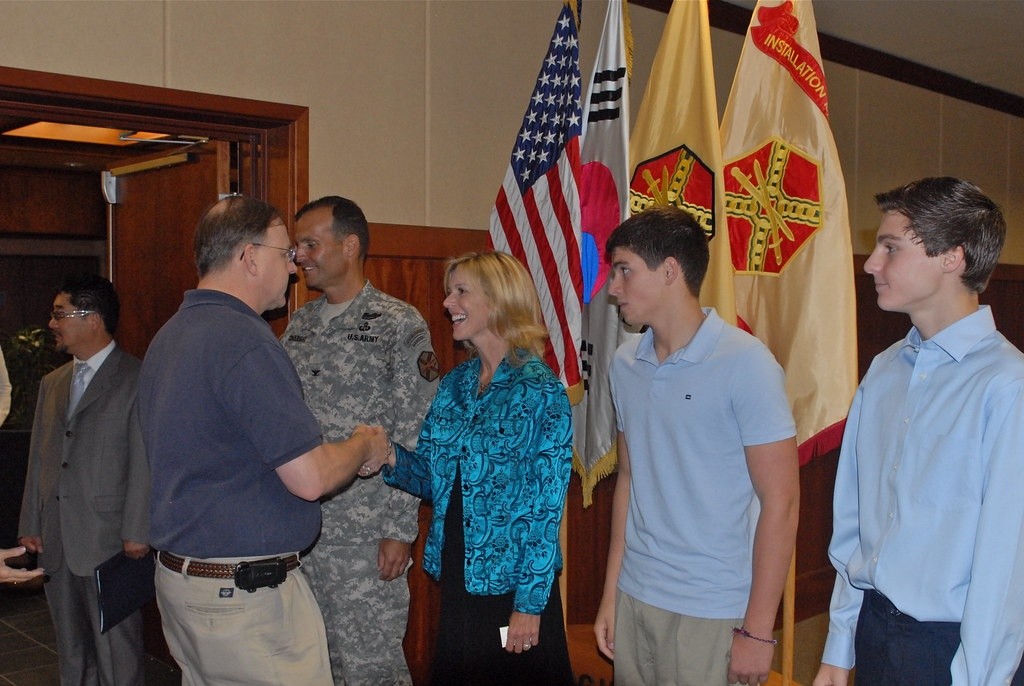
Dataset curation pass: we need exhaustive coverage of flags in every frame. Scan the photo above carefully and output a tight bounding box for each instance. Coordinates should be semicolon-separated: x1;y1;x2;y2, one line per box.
630;0;735;326
486;0;630;648
721;0;858;686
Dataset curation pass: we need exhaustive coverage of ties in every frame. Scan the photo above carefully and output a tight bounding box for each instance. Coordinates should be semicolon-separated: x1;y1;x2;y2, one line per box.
67;363;91;420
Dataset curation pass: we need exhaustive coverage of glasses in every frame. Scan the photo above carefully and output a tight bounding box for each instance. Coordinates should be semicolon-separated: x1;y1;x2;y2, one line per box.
240;243;297;266
51;311;92;321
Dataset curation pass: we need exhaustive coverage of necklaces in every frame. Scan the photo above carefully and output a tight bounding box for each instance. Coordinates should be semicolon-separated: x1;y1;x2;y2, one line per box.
480;383;487;393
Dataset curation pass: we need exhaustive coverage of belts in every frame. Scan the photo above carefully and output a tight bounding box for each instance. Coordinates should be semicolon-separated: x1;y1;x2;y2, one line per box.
159;550;301;579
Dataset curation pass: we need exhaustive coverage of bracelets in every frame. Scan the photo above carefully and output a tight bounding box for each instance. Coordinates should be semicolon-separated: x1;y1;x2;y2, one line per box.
385;440;392;461
731;627;778;646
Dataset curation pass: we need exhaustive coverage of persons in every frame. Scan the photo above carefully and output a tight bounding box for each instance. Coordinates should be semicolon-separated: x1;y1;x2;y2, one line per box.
0;546;43;584
17;275;150;686
280;195;438;686
813;174;1024;686
352;253;575;686
594;206;800;686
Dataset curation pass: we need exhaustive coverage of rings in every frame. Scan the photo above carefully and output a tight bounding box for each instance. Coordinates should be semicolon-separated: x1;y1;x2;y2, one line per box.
365;471;368;474
367;466;371;471
14;581;17;584
522;642;531;649
361;465;365;468
